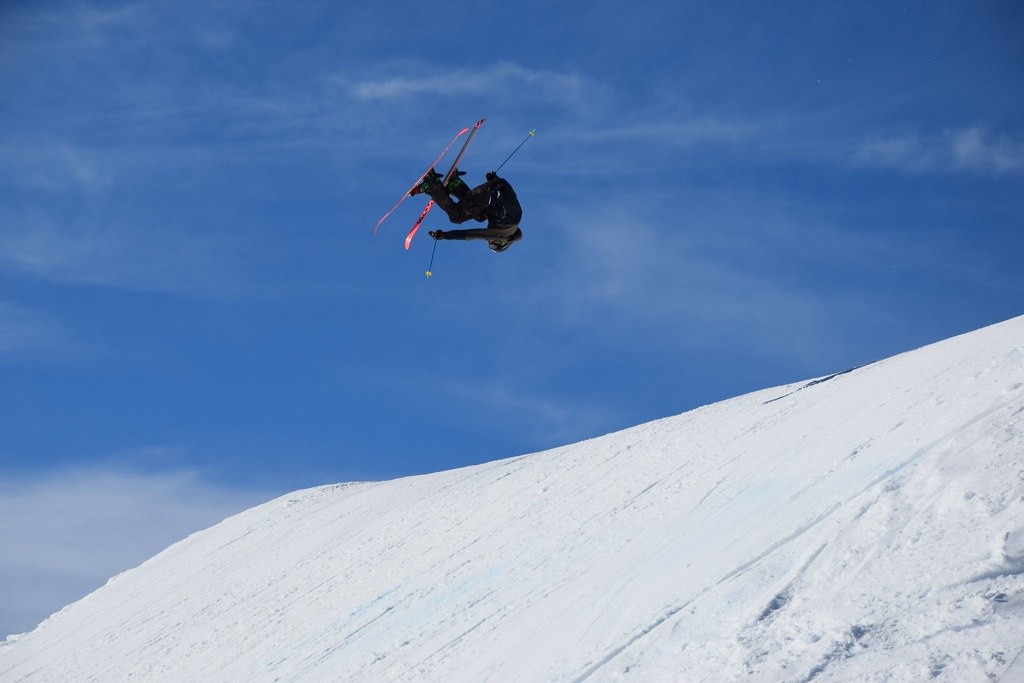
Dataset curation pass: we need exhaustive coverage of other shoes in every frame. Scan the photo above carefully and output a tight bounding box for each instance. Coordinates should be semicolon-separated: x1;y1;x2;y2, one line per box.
412;169;439;195
446;172;463;195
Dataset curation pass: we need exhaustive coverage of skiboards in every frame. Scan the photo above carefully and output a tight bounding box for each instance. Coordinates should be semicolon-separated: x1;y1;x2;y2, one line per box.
372;117;488;250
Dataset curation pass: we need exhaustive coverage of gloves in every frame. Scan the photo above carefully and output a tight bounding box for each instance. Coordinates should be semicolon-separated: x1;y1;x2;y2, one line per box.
486;171;497;180
435;230;445;240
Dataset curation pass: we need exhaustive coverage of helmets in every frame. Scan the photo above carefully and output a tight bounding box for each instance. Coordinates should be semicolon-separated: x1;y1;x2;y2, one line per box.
491;243;510;253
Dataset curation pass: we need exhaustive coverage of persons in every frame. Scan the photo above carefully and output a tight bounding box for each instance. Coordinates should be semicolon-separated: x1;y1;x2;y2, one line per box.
417;167;522;252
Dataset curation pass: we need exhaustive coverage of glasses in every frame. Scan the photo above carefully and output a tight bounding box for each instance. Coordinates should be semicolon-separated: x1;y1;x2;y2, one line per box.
488;240;500;250
